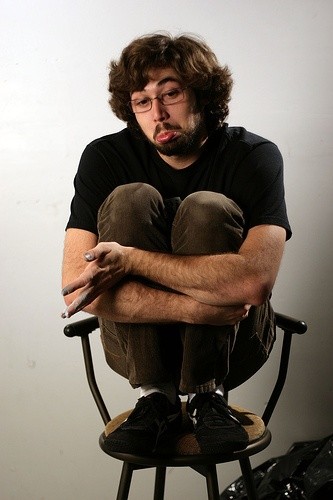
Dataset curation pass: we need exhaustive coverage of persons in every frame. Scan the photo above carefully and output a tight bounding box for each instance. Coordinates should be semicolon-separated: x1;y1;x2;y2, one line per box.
60;30;293;454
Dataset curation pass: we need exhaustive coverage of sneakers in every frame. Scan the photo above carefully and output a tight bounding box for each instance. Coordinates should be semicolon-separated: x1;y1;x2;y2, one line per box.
104;391;182;458
186;392;248;453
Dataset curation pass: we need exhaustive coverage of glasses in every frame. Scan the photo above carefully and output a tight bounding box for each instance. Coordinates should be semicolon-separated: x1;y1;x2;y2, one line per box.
126;85;190;113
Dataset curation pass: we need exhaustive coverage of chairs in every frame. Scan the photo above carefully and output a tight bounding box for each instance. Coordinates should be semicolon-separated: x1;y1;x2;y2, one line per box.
63;309;308;500
